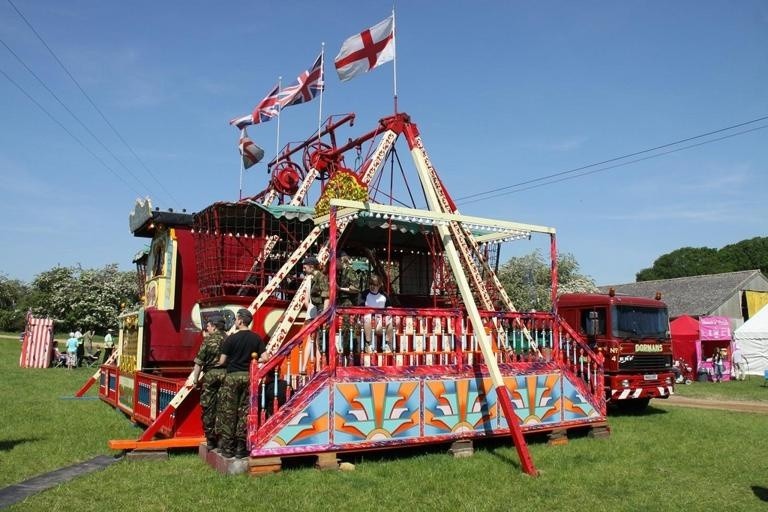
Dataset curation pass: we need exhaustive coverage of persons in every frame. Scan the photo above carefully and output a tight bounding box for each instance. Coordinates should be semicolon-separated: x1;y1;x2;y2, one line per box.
218;309;266;459
52;326;115;369
731;347;749;381
672;361;680;379
347;276;393;354
711;347;724;384
301;257;330;366
336;252;363;367
193;317;228;453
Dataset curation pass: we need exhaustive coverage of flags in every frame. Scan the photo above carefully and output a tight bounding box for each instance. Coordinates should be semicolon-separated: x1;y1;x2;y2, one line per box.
334;13;394;83
239;128;264;170
228;84;280;130
279;51;324;111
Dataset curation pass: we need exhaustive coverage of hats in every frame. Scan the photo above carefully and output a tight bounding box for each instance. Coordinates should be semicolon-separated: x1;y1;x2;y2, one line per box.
302;257;318;264
207;316;226;323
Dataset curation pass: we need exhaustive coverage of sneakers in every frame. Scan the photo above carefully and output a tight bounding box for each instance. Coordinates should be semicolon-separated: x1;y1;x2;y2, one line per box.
206;440;247;458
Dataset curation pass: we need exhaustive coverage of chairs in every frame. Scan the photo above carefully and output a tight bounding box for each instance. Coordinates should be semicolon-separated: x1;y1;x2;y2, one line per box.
51;350;100;367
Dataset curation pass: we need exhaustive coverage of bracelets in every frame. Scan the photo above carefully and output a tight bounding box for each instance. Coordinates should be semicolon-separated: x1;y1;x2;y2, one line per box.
194;381;199;384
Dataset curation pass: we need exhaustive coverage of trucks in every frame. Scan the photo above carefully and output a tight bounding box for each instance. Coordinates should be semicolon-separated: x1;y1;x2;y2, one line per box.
504;287;675;412
